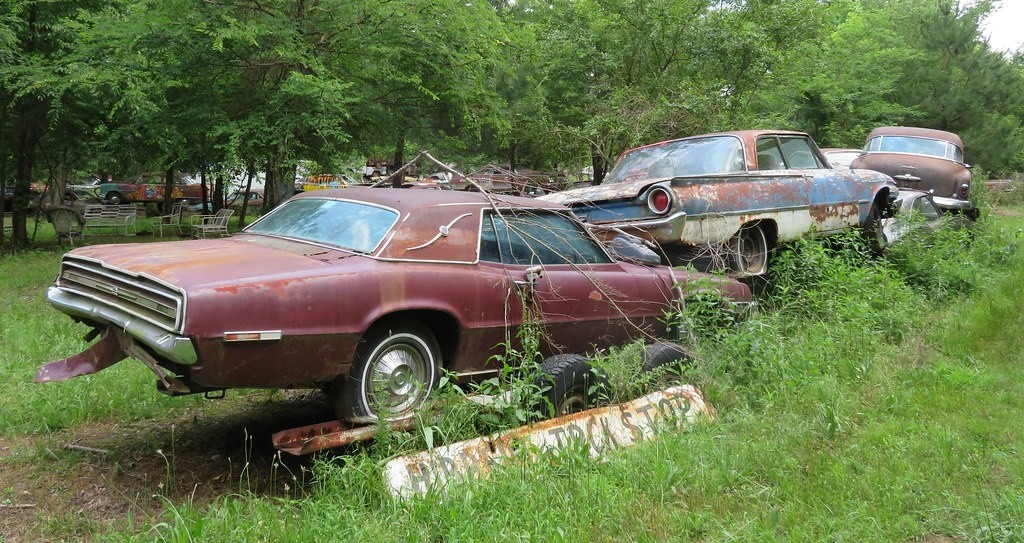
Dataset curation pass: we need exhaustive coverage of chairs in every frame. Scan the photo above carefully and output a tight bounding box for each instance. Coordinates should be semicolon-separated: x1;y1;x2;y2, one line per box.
46;205;86;246
151;203;183;237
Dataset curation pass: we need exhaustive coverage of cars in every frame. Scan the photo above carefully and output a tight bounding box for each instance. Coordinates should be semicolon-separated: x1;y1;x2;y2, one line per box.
46;182;752;427
778;148;866;171
1;162;569;228
852;125;980;222
537;131;900;280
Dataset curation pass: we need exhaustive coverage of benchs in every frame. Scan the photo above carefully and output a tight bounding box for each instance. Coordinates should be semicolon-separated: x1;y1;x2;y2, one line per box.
190;209;235;239
73;204;138;237
